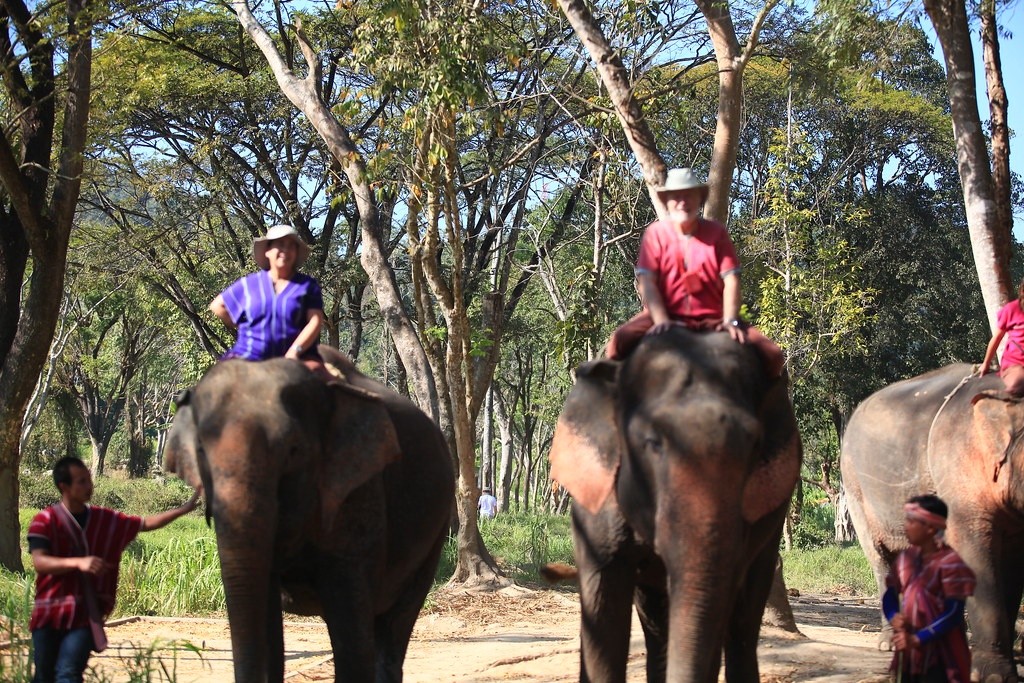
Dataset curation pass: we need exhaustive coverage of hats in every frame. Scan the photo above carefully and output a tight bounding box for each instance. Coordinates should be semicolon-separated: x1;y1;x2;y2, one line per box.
252;225;310;270
656;168;709;208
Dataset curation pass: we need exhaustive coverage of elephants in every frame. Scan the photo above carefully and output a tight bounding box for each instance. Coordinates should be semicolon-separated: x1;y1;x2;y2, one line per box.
837;361;1024;683
546;324;804;683
163;342;460;683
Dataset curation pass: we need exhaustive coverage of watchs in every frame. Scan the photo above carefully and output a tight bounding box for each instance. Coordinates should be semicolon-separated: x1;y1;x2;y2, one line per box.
292;344;303;353
724;319;739;326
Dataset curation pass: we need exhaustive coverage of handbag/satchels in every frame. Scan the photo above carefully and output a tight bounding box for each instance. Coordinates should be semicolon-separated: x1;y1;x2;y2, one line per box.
86;584;107;653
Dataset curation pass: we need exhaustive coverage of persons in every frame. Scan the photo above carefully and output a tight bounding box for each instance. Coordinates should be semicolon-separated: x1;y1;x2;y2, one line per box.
605;167;785;377
882;495;977;683
27;455;204;683
477;487;497;525
209;225;334;382
974;277;1024;396
